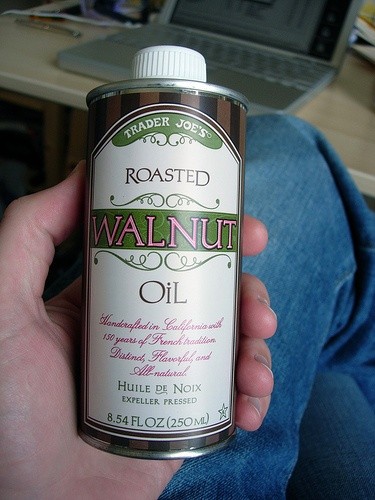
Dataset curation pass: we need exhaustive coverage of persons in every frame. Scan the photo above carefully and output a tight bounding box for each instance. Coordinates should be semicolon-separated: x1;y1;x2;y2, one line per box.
0;111;374;500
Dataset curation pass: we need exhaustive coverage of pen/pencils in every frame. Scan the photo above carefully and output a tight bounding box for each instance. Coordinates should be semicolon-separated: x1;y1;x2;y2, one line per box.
14;18;82;38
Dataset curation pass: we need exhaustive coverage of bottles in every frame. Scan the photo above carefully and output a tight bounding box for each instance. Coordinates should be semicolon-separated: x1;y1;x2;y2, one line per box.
76;43;250;461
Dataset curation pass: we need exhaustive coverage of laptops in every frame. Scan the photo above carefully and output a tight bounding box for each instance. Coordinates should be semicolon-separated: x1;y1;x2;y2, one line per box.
53;0;365;117
